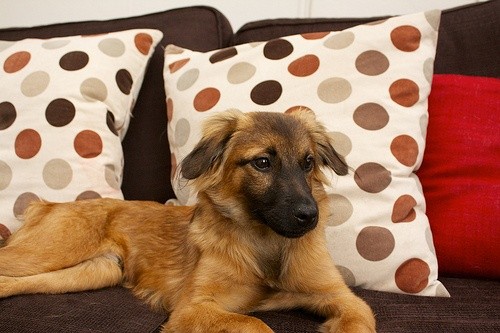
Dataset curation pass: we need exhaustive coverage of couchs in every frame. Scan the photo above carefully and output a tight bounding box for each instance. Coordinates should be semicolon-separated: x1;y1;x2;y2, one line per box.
0;0;500;333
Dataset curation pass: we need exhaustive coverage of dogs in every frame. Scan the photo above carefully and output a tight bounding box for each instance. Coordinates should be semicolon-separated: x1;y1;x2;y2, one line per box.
0;107;377;333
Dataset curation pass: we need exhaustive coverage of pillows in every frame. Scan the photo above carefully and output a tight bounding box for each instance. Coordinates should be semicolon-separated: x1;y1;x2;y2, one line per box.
0;28;164;246
163;9;451;297
414;74;500;277
